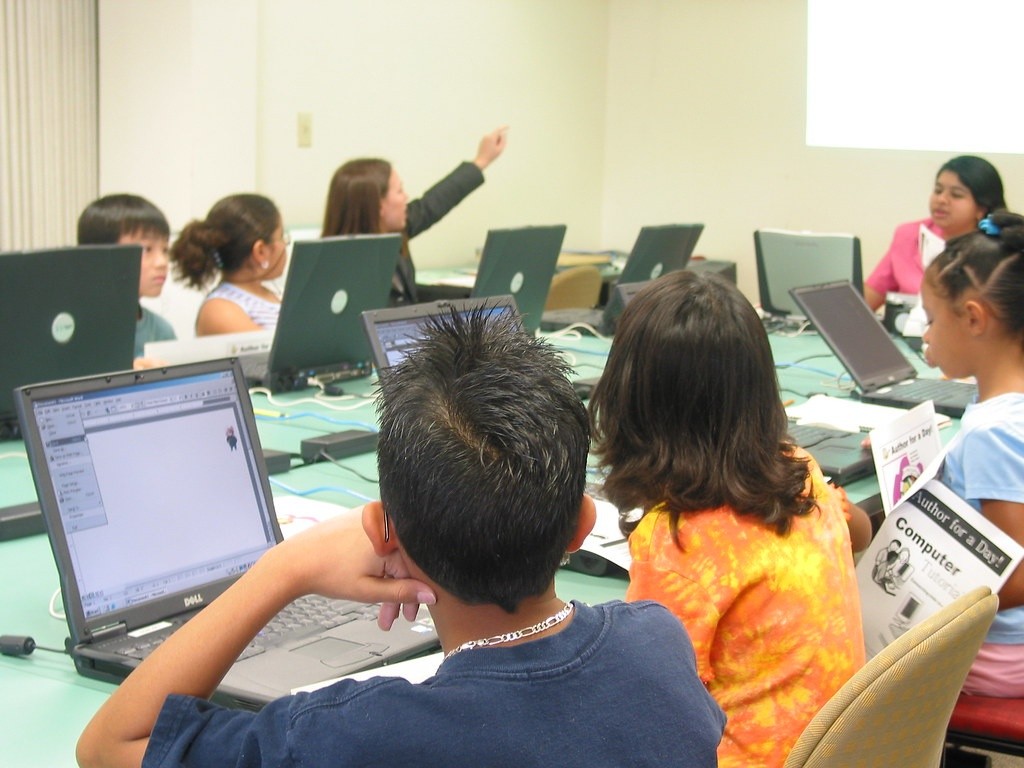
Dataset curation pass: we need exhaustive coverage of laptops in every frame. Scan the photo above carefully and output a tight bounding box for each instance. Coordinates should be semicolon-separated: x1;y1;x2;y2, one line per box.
1;223;980;710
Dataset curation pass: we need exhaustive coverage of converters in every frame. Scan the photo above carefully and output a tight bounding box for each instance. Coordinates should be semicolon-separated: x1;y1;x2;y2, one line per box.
300;429;378;464
261;448;292;474
573;376;603;399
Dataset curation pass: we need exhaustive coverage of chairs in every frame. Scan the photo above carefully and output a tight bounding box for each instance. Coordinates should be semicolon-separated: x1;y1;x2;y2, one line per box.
785;588;1001;767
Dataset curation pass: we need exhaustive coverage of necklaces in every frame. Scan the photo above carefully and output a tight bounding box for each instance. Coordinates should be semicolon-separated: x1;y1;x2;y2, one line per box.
447;602;574;655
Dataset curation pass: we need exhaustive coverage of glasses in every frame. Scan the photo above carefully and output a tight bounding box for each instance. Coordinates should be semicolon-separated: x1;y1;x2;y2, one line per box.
262;230;291;246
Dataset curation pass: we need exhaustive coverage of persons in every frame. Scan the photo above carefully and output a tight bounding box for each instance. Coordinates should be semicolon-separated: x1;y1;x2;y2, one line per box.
172;194;287;335
592;270;873;767
74;299;727;768
320;125;508;305
77;195;178;370
865;156;1006;312
862;210;1023;697
